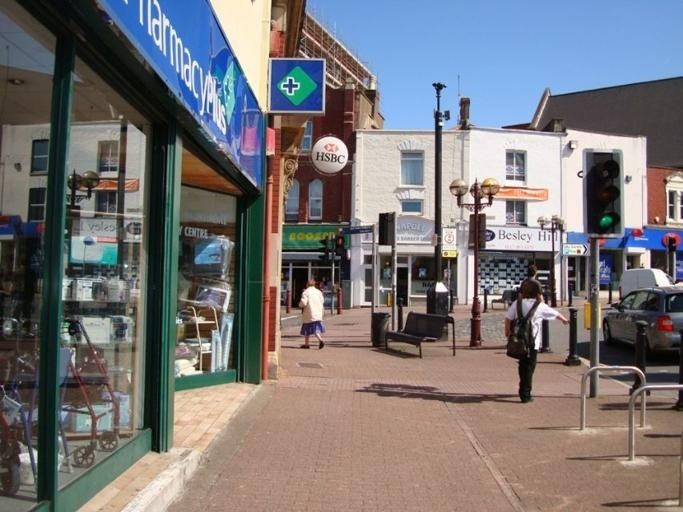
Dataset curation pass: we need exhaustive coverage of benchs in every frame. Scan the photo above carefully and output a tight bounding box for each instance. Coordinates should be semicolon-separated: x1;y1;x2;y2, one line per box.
382;308;456;362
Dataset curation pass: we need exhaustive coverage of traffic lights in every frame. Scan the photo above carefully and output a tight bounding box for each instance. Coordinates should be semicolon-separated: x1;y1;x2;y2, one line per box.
319;237;329;262
585;149;622;235
669;237;677;251
335;235;344;255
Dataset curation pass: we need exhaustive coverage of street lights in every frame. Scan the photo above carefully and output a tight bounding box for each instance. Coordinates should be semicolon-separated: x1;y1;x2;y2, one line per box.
448;176;500;350
426;80;452;339
537;215;566;310
63;170;98;316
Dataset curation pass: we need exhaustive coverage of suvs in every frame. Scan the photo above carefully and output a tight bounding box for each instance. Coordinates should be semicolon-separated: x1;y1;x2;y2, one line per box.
602;283;683;361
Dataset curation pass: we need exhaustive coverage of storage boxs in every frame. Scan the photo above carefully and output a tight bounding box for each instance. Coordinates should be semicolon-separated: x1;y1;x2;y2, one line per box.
74;314;112;347
71;405;111;433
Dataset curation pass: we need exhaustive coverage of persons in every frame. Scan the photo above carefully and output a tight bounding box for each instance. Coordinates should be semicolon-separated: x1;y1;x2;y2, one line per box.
299;277;325;350
504;279;569;403
515;265;545;301
317;275;327;291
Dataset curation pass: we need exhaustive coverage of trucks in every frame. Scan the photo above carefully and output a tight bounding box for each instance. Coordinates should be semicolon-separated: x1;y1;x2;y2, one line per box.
618;267;675;304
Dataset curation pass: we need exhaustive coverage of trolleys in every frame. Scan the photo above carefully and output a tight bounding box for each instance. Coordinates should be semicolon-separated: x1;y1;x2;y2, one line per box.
13;319;120;468
0;407;22;498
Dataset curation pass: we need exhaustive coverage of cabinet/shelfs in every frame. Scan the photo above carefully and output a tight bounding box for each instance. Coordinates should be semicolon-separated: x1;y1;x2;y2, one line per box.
178;302;219;374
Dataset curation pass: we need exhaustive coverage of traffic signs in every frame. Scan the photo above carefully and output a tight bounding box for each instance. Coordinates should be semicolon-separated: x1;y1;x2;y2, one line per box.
342;226;374;235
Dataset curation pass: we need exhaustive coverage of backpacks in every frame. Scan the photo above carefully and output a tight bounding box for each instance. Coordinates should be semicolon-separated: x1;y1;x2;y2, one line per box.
507;299;541;358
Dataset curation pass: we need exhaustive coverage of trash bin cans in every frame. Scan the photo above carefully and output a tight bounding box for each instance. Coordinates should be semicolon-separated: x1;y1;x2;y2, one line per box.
371;312;391;347
